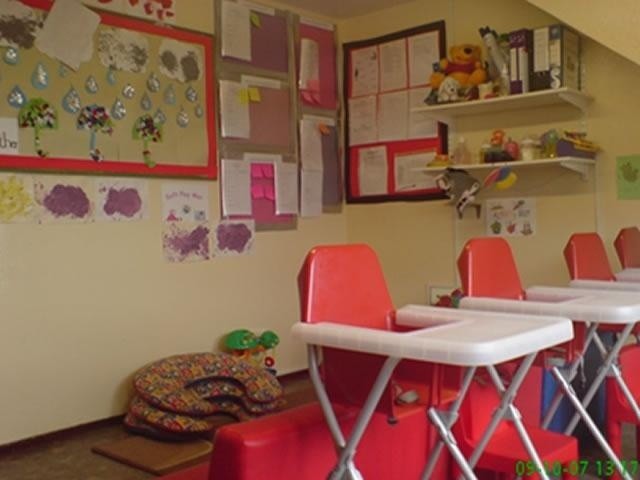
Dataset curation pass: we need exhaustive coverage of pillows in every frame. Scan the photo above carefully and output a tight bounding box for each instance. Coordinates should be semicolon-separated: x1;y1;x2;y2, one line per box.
121;351;289;443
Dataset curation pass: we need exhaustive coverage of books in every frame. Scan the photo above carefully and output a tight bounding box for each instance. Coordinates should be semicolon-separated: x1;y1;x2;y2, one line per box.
477;22;582;94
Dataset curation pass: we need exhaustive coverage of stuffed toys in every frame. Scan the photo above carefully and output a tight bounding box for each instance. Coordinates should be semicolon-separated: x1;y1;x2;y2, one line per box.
438;76;460;103
435;167;483;220
430;41;488;92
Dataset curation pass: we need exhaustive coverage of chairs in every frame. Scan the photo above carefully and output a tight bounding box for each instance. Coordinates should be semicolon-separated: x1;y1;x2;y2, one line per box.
562;228;639;478
291;242;576;479
455;236;640;480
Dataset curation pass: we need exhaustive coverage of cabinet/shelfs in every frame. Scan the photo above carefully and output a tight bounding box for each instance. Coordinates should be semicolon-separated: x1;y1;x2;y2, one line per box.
407;86;593;184
